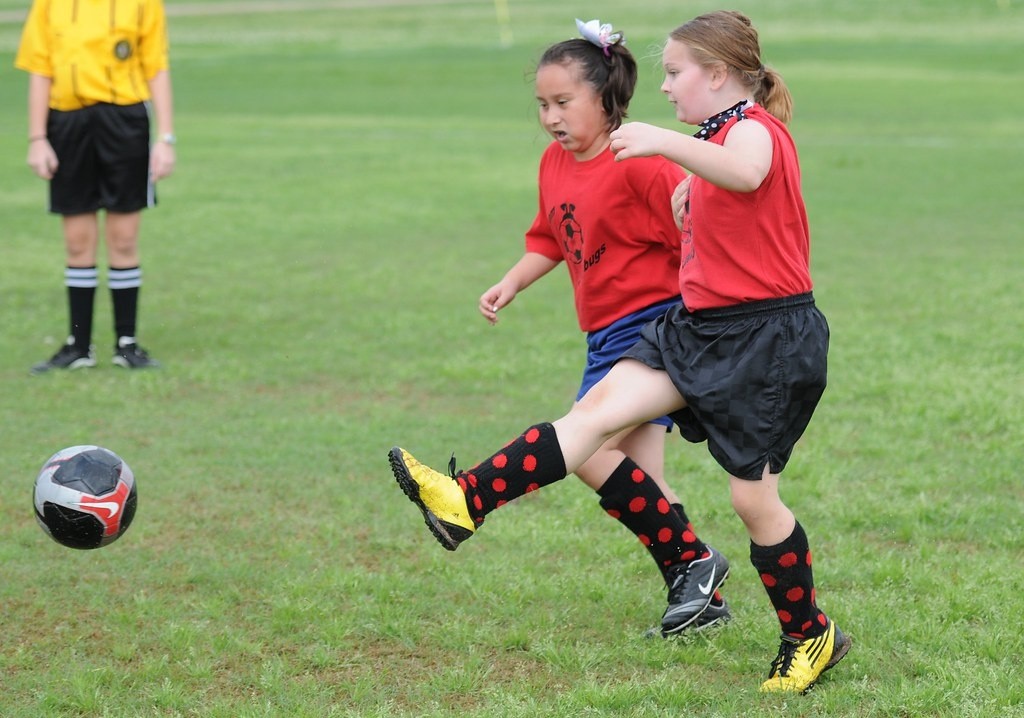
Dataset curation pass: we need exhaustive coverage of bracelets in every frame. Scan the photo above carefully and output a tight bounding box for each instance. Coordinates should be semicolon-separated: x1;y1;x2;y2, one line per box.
28;136;47;143
158;134;176;144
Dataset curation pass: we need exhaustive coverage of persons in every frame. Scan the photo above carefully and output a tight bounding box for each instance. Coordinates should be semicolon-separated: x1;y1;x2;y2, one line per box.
388;9;852;696
478;18;731;644
13;0;177;377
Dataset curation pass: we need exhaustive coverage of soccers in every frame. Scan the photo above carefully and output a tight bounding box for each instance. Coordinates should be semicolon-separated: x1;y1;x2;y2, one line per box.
33;445;139;550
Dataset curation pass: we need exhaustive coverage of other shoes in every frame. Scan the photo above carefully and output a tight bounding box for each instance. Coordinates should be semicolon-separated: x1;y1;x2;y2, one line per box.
28;344;96;376
111;345;161;369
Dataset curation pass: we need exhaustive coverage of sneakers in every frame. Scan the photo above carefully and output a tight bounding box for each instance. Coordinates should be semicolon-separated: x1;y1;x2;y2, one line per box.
661;543;731;634
387;446;484;551
760;607;851;696
640;597;731;639
